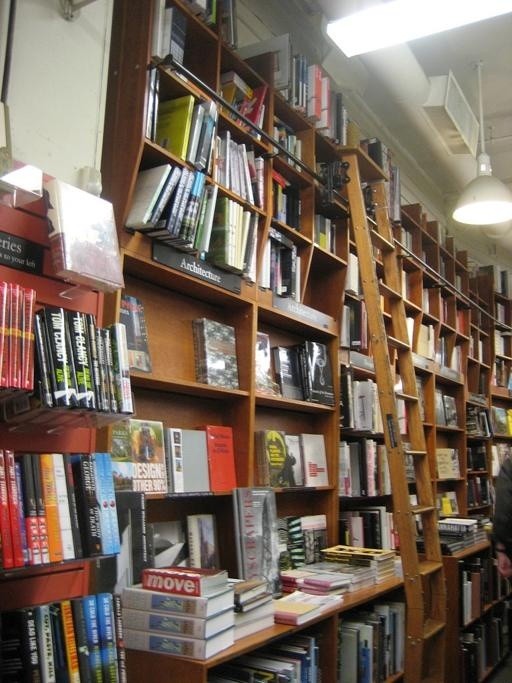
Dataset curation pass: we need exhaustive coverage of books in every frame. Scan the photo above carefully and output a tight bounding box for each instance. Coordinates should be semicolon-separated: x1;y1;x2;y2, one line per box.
118;293;406;683
1;154;135;683
348;137;511;436
401;438;512;683
123;1;347;301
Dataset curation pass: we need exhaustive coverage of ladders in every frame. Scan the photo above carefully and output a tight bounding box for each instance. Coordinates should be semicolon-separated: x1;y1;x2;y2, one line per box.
336;144;447;683
